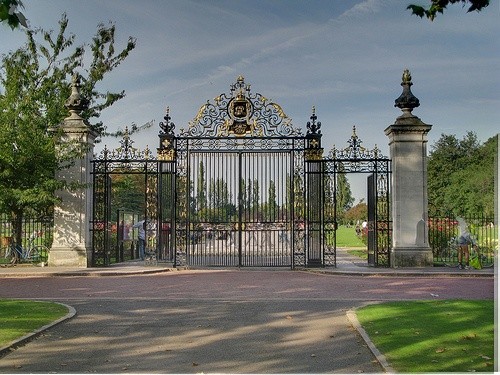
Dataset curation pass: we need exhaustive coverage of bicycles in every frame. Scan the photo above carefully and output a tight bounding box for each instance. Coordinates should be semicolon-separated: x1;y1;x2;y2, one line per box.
441;237;494;268
0;231;50;268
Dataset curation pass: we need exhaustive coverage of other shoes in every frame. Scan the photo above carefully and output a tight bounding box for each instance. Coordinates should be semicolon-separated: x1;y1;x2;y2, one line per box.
465;266;472;270
456;265;462;270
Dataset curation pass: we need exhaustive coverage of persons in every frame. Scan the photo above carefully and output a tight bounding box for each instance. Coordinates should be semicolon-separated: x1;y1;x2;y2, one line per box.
132;215;147;261
194;220;290;249
450;217;479;269
346;220;367;239
